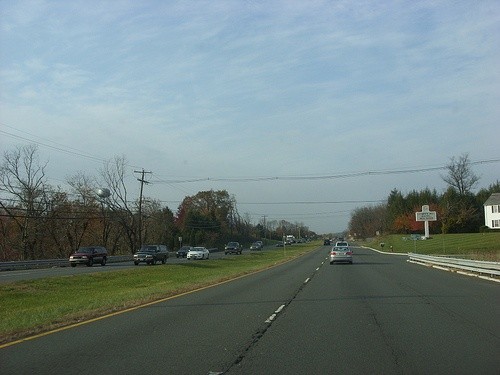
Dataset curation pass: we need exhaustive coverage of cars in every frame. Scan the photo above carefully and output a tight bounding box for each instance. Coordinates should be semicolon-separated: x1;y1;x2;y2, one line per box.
328;246;354;264
187;247;209;260
296;237;311;243
176;246;193;258
285;239;291;245
276;241;284;247
250;243;261;250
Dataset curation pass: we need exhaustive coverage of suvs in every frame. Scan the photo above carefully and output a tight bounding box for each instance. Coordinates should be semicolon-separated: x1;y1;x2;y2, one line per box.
133;245;169;266
256;241;263;248
324;239;330;245
224;242;242;255
68;246;109;267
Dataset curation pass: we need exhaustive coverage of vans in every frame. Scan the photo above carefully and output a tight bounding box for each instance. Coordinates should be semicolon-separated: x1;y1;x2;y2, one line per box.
336;241;350;248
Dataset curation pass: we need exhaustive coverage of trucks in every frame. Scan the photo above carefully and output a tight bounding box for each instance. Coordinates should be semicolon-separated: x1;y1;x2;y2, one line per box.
287;235;295;244
